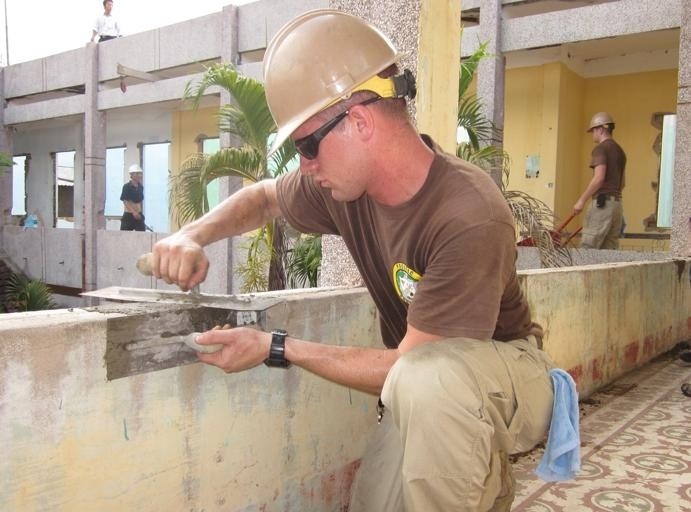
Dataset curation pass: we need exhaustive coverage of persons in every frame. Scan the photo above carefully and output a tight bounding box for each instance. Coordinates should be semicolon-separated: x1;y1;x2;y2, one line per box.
149;10;555;512
571;110;628;251
120;165;147;232
87;1;123;43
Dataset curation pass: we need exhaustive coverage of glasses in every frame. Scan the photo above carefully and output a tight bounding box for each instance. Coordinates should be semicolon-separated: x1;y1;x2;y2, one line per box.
294;97;379;160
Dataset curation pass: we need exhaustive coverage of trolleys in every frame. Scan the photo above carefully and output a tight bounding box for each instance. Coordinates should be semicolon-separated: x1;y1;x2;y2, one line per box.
516;206;584;248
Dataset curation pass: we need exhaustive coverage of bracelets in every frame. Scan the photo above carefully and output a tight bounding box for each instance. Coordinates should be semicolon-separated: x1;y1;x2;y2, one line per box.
264;326;292;370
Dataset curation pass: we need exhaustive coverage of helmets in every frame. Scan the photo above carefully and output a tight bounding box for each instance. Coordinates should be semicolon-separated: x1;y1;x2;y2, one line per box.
586;111;615;133
129;165;142;173
263;9;417;159
